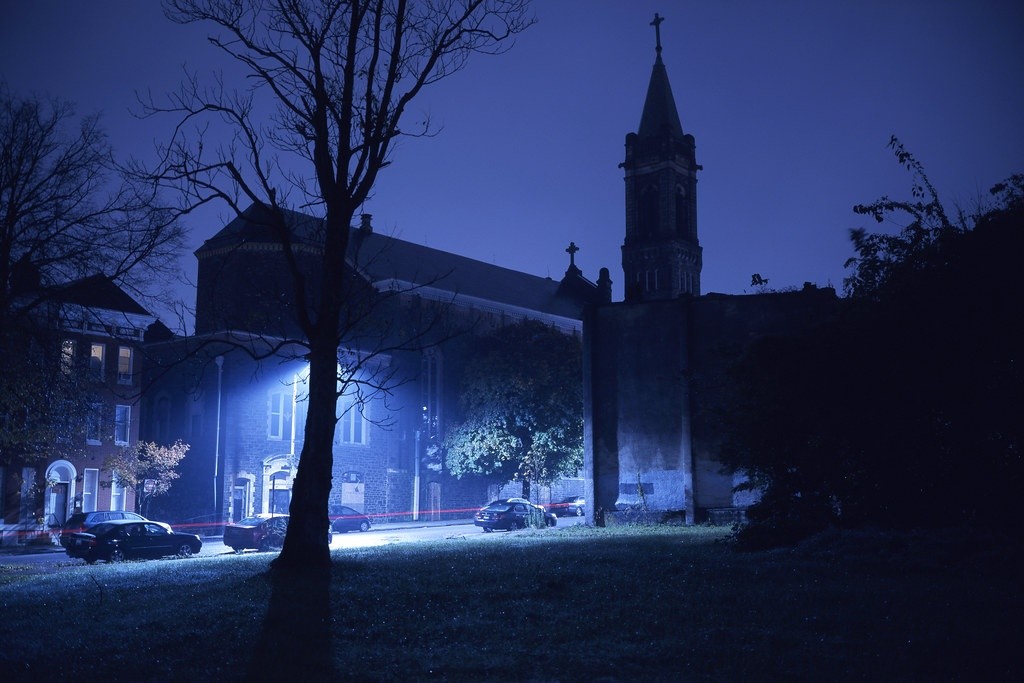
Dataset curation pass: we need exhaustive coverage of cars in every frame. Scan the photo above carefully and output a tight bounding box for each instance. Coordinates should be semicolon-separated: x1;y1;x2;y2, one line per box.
328;504;372;534
551;496;585;517
473;497;558;533
65;519;203;564
223;513;290;552
59;509;173;548
